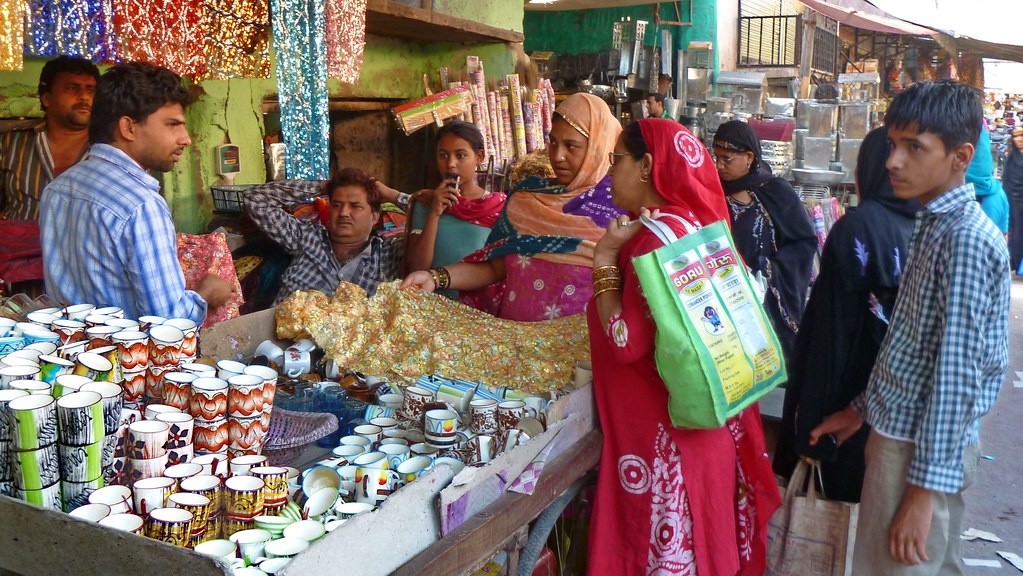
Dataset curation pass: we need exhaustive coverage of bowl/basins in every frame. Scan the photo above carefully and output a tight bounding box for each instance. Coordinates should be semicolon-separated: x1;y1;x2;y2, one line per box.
790;168;847;185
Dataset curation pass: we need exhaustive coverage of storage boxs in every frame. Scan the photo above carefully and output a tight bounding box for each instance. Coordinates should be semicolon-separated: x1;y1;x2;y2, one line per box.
210;185;249;212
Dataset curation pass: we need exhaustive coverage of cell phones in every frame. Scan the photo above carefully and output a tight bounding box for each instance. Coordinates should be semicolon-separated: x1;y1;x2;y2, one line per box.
444;173;460;206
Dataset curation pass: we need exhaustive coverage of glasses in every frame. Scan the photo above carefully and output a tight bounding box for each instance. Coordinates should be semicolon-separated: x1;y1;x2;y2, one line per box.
710;153;740;164
609;152;643;165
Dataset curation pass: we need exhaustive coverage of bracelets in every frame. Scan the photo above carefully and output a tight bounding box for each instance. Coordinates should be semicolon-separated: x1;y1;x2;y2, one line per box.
590;266;622;298
425;266;451;291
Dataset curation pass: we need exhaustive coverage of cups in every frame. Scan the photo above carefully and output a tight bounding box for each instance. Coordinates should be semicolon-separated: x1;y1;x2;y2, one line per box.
0;293;592;576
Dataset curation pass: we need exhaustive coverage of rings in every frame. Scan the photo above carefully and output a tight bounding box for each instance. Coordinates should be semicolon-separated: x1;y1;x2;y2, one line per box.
618;221;628;225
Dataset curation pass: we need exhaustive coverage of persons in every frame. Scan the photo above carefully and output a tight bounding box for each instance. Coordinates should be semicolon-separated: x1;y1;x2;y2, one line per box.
0;55;101;223
646;93;674;120
39;59;235;338
405;120;509;302
401;92;633;322
585;118;785;576
713;79;1022;576
241;169;411;309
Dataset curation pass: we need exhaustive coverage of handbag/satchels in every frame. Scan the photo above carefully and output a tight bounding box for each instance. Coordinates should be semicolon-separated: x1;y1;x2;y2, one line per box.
631;212;788;428
765;457;851;576
